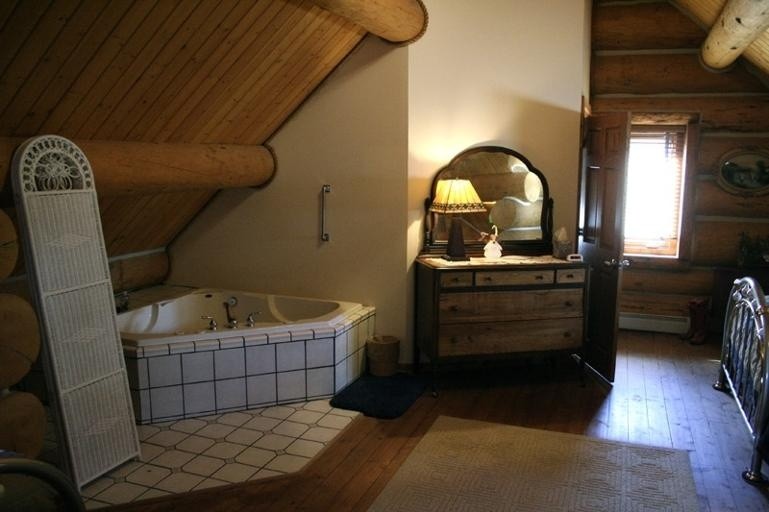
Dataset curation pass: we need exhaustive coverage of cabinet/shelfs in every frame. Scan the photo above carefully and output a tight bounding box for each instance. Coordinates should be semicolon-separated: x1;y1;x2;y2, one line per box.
412;257;591;398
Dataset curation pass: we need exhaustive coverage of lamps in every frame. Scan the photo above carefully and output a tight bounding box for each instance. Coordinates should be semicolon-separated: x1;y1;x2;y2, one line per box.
429;179;488;261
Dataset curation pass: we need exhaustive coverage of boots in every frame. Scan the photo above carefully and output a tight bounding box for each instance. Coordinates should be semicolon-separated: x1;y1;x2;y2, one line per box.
679;298;711;346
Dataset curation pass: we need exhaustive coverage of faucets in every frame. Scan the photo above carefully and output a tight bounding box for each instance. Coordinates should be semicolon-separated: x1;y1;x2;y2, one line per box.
222;299;238;330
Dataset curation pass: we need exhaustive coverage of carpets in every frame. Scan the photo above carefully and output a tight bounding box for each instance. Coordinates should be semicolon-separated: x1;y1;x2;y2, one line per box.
330;370;427;419
366;414;699;512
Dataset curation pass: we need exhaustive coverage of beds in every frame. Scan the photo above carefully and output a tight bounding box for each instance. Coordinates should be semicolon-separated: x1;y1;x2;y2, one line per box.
712;277;769;486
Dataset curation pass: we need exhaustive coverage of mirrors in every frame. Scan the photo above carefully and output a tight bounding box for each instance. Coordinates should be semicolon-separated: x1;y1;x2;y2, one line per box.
424;145;554;256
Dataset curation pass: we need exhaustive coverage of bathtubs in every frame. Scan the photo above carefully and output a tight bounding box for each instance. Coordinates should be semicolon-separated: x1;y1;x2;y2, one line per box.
117;284;378;423
